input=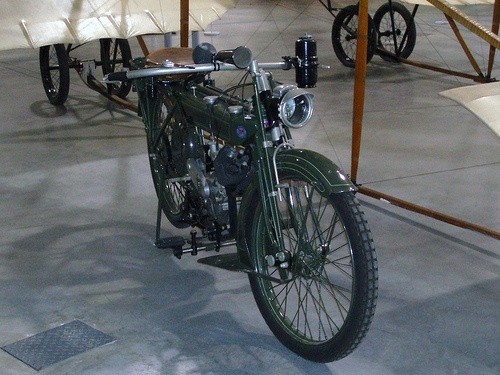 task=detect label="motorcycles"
[100,32,380,362]
